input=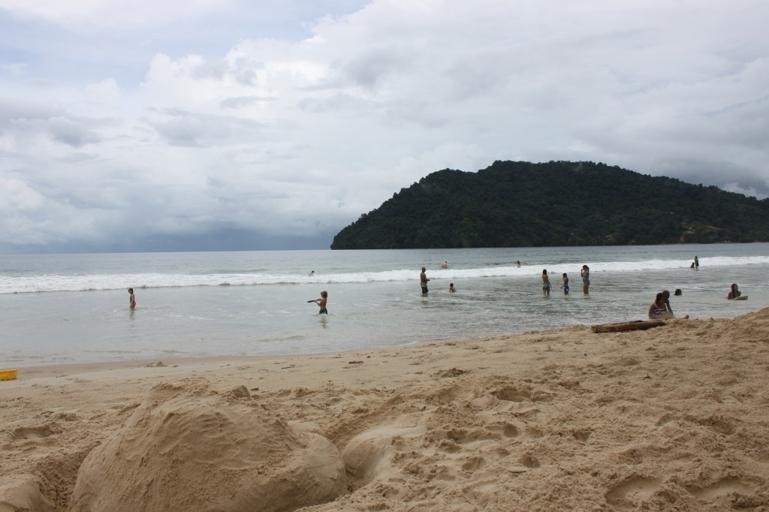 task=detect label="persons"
[559,272,569,296]
[689,256,699,269]
[541,268,552,298]
[441,259,448,268]
[449,282,456,293]
[128,288,136,312]
[580,265,590,295]
[308,271,316,277]
[314,291,328,315]
[727,283,741,300]
[420,266,431,296]
[516,260,521,267]
[674,289,682,296]
[648,290,673,318]
